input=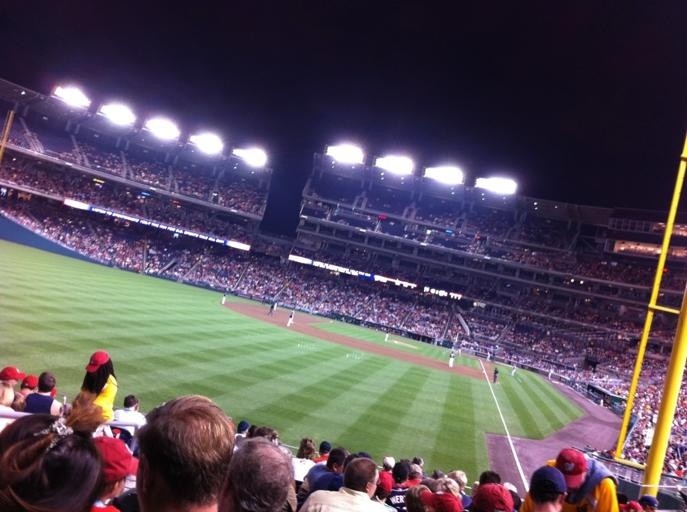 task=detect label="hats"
[420,483,515,512]
[556,448,587,475]
[0,366,26,381]
[90,435,140,483]
[23,375,39,388]
[529,464,568,495]
[639,495,659,508]
[383,456,396,469]
[85,350,111,373]
